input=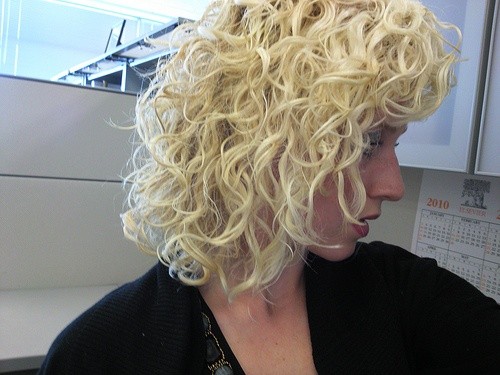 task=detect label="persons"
[36,0,500,375]
